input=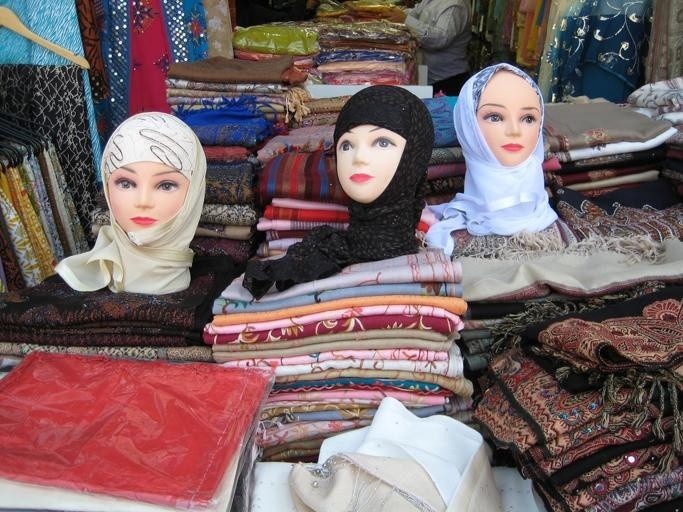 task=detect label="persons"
[424,62,574,260]
[53,111,206,296]
[375,0,474,98]
[241,84,437,301]
